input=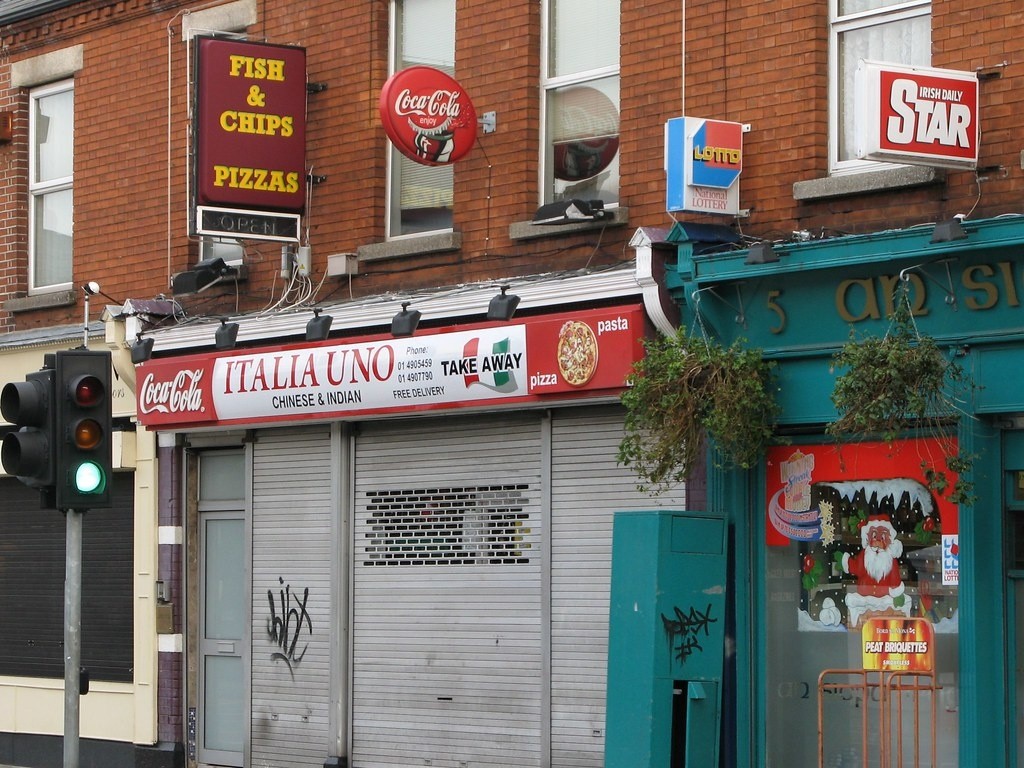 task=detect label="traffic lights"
[1,370,54,488]
[56,350,114,511]
